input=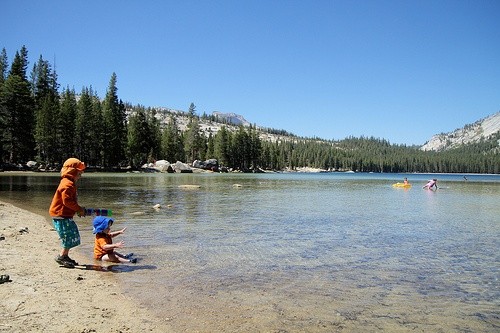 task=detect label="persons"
[48,157,87,269]
[423,178,437,190]
[404,177,408,184]
[92,216,137,264]
